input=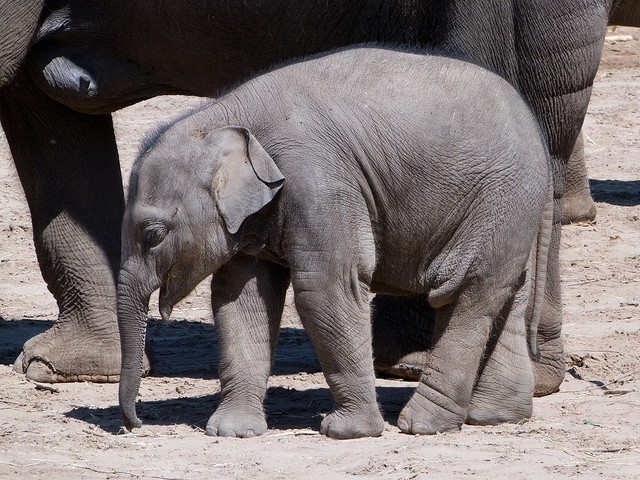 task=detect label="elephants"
[118,42,555,435]
[0,2,613,383]
